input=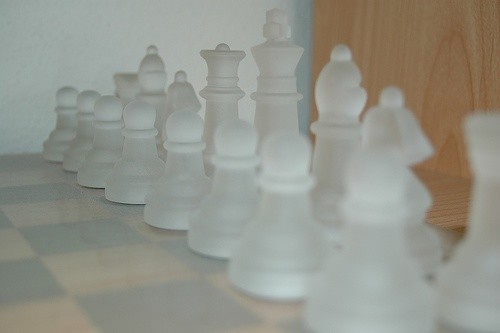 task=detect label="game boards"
[0,152,312,333]
